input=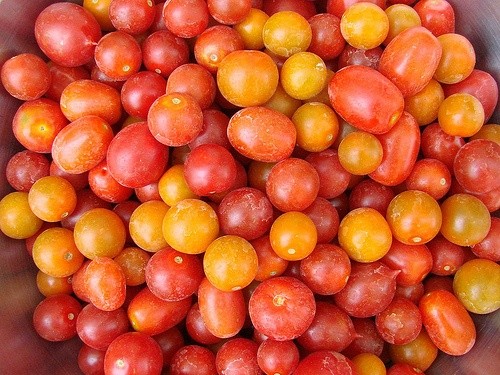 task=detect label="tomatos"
[0,0,500,375]
